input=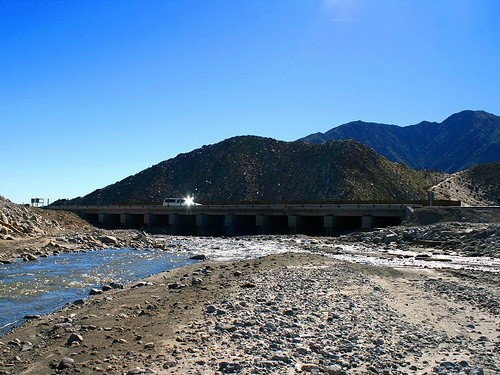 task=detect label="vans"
[163,198,202,206]
